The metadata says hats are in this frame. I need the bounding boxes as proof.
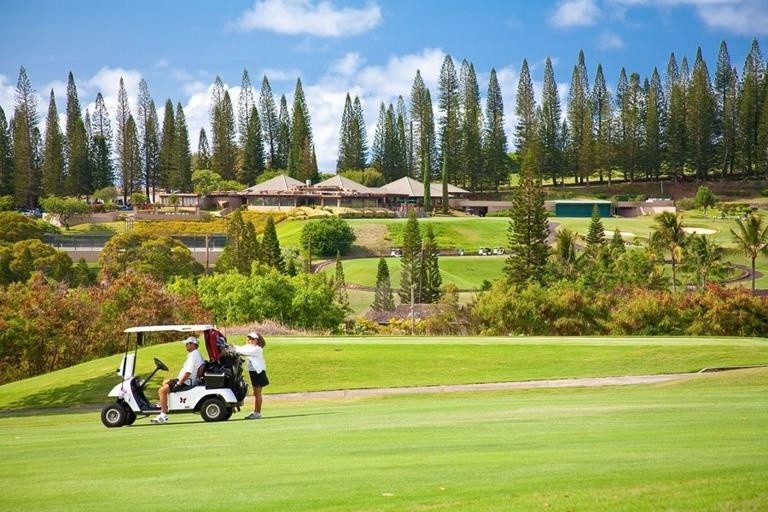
[89,198,132,209]
[21,205,46,219]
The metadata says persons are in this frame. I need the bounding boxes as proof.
[150,336,204,424]
[229,332,269,420]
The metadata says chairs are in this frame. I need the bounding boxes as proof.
[244,412,261,419]
[150,414,168,424]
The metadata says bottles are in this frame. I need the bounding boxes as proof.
[247,331,259,339]
[181,336,199,346]
